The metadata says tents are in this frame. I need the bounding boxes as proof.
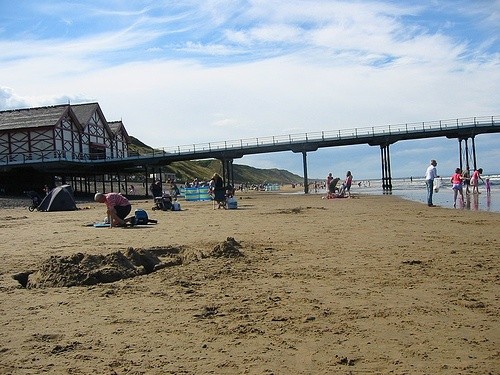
[38,185,77,212]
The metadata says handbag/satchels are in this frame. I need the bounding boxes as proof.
[435,183,439,193]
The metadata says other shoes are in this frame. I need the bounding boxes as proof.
[429,204,436,207]
[118,222,127,227]
[130,216,136,227]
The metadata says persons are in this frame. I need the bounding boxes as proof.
[451,168,469,207]
[326,173,333,192]
[345,171,353,199]
[340,183,347,195]
[185,177,209,188]
[94,192,131,229]
[424,159,440,207]
[464,166,471,194]
[210,173,227,210]
[169,181,180,201]
[485,178,490,193]
[470,168,484,194]
[328,178,340,193]
